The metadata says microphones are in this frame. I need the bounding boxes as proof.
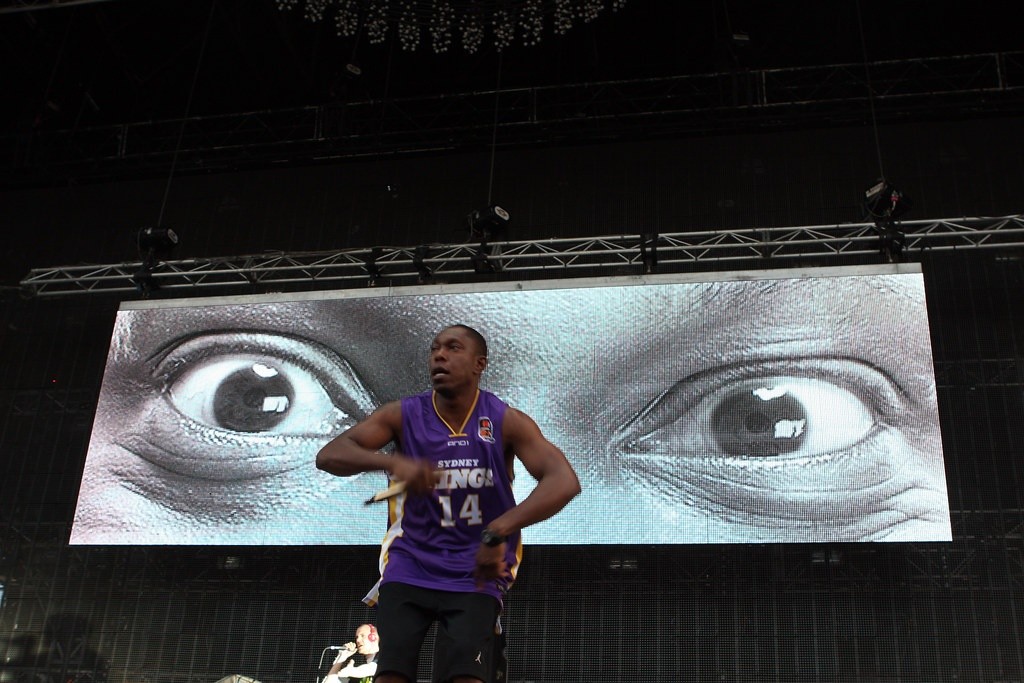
[364,480,408,505]
[326,646,348,650]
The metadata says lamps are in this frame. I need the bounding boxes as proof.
[861,176,911,255]
[136,225,178,267]
[466,205,510,243]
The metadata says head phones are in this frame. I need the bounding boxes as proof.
[368,624,377,642]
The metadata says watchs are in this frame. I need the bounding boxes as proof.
[480,526,506,547]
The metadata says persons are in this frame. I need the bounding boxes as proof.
[316,325,581,683]
[68,262,953,544]
